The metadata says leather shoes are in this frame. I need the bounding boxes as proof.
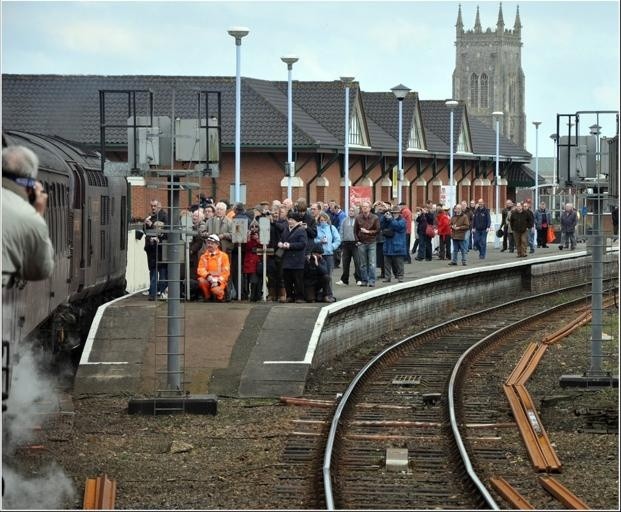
[399,278,403,282]
[383,278,391,282]
[415,257,423,260]
[369,283,375,286]
[361,281,367,286]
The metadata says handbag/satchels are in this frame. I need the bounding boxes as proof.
[547,227,556,243]
[426,224,435,237]
[225,279,237,301]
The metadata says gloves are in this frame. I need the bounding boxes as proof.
[278,241,284,249]
[284,242,290,250]
[207,274,218,283]
[211,282,219,288]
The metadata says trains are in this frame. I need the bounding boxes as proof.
[1,131,131,413]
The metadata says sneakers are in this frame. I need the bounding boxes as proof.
[336,280,347,286]
[448,262,457,265]
[501,248,506,252]
[356,280,362,286]
[143,290,150,296]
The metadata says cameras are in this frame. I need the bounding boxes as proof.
[386,212,390,215]
[17,177,43,190]
[265,210,273,216]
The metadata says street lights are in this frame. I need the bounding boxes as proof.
[279,56,298,200]
[491,111,504,224]
[389,83,412,203]
[548,133,560,219]
[443,101,459,218]
[339,76,355,217]
[224,26,250,205]
[590,124,602,178]
[531,121,543,211]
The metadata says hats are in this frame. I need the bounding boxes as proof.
[392,206,401,212]
[208,234,221,245]
[288,212,299,222]
[253,204,263,214]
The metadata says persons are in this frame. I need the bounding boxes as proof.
[334,198,412,286]
[142,199,169,301]
[180,192,342,302]
[560,203,580,252]
[533,201,552,248]
[410,199,491,265]
[2,147,55,290]
[500,200,535,259]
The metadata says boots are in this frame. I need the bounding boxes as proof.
[268,287,276,301]
[280,287,286,302]
